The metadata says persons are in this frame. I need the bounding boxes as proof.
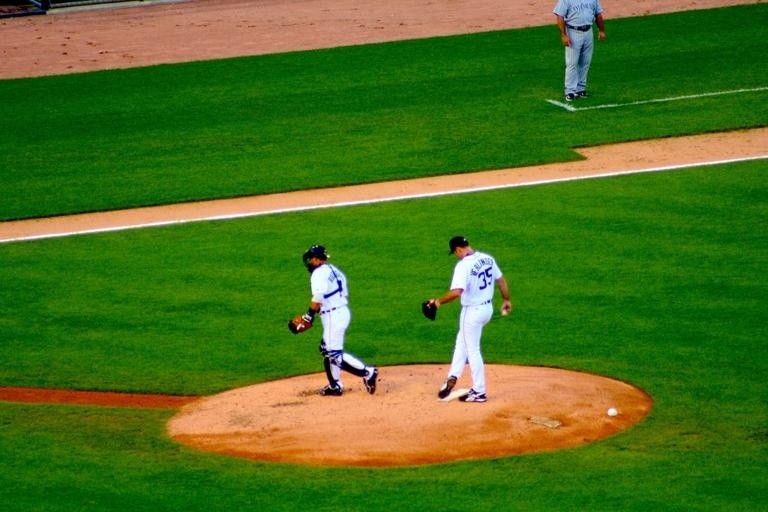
[421,236,512,403]
[288,244,379,396]
[552,0,608,102]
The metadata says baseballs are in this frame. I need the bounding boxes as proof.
[608,408,617,417]
[502,310,508,316]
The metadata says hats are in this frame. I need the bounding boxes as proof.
[447,236,468,255]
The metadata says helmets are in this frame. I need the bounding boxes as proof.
[303,245,327,272]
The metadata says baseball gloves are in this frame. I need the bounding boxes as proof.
[422,298,440,320]
[288,314,313,334]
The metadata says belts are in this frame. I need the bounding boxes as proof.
[565,23,590,32]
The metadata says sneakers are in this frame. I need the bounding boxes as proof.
[459,388,487,402]
[320,384,343,395]
[565,91,590,102]
[439,375,457,399]
[363,366,378,394]
